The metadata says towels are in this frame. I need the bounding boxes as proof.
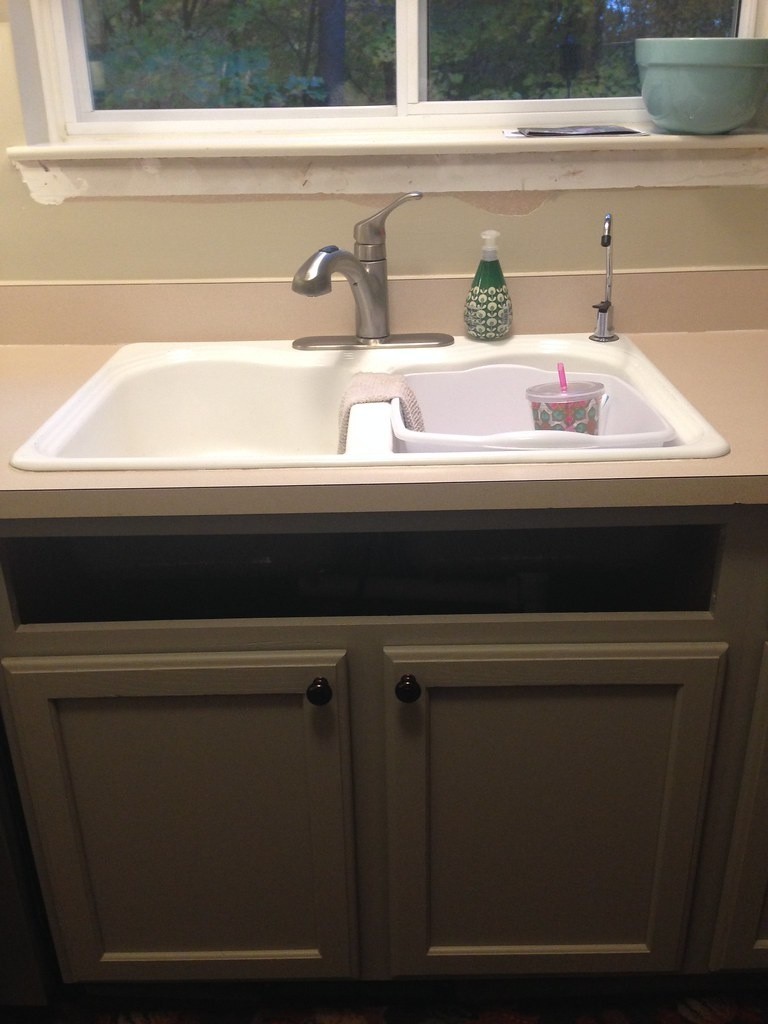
[336,371,427,455]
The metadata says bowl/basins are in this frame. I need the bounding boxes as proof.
[633,36,768,136]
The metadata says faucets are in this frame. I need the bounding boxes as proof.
[590,211,617,338]
[292,191,424,348]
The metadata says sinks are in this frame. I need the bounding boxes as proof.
[51,354,357,460]
[389,349,690,456]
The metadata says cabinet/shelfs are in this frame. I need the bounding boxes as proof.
[1,506,767,991]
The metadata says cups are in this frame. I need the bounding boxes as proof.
[526,378,610,440]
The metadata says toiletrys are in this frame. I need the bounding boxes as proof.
[461,227,514,341]
